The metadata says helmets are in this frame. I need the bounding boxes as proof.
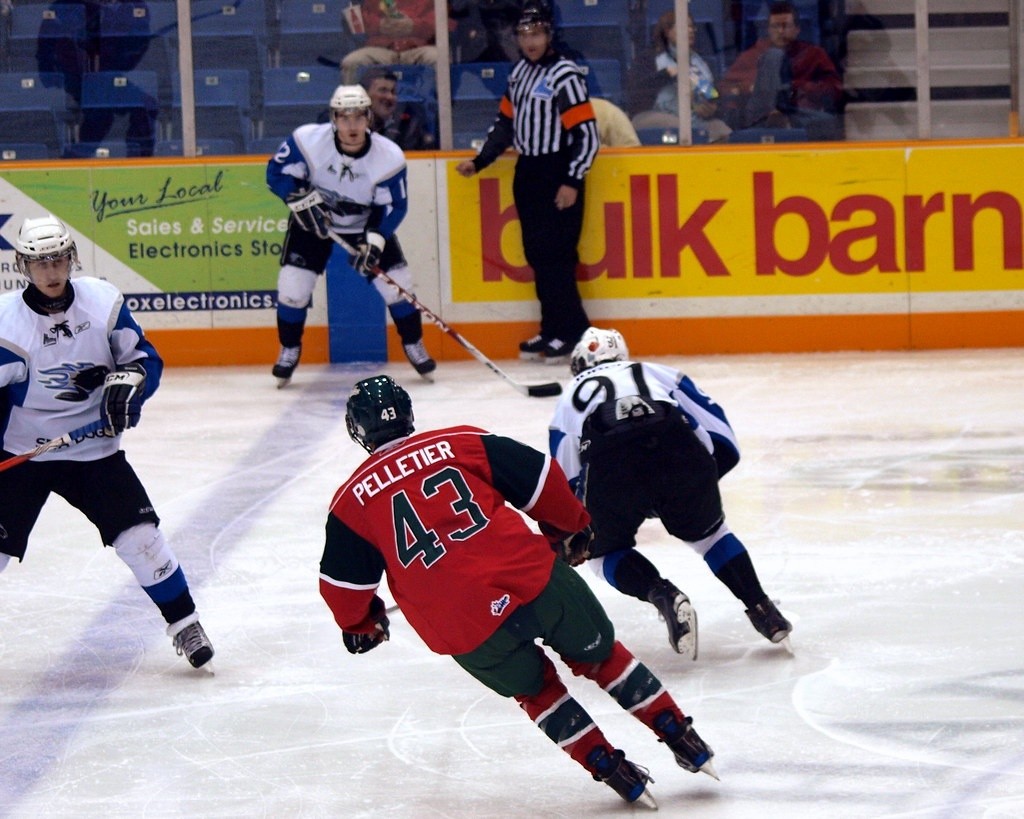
[509,1,552,32]
[330,84,372,108]
[14,215,75,261]
[570,327,629,376]
[345,375,414,456]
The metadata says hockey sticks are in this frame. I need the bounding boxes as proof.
[386,605,399,614]
[0,418,108,471]
[327,227,563,398]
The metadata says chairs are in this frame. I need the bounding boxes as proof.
[0,0,842,163]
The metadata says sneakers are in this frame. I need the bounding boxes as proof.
[545,337,577,365]
[648,579,699,661]
[742,597,795,655]
[402,338,437,383]
[518,334,553,358]
[165,610,216,678]
[653,707,721,781]
[596,753,657,811]
[272,342,303,389]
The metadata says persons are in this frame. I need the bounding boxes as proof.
[0,214,216,674]
[586,97,642,149]
[317,375,719,810]
[457,3,600,366]
[547,327,794,659]
[340,0,457,82]
[626,12,732,142]
[266,86,437,387]
[718,5,848,130]
[318,69,437,152]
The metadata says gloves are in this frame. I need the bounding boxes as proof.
[342,595,391,655]
[99,362,146,437]
[538,523,595,565]
[287,189,334,239]
[349,233,387,279]
